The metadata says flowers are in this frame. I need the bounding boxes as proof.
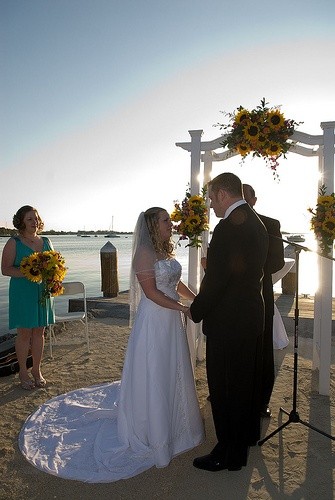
[306,183,335,257]
[19,250,69,305]
[211,95,306,185]
[169,180,211,250]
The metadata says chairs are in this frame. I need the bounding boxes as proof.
[47,281,91,358]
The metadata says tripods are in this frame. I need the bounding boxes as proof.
[257,234,335,446]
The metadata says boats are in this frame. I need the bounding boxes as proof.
[94,236,98,237]
[77,235,91,237]
[287,235,307,242]
[125,237,128,238]
[104,232,120,238]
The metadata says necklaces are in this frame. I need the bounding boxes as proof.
[23,235,39,243]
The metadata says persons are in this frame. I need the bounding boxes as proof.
[185,171,272,473]
[1,205,55,390]
[201,183,285,417]
[116,206,206,470]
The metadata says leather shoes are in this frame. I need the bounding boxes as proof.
[193,454,242,472]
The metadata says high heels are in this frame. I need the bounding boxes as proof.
[18,369,47,389]
